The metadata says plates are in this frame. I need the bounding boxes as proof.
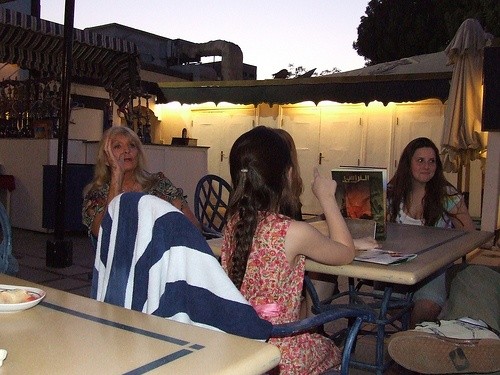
[0,283,47,312]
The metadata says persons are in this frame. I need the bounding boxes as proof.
[387,265,500,375]
[219,126,383,375]
[305,138,476,375]
[82,126,202,255]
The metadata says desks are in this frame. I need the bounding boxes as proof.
[0,273,281,375]
[204,218,495,375]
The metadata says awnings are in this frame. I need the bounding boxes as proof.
[156,70,454,106]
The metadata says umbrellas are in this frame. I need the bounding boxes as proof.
[440,19,492,197]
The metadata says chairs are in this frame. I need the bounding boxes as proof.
[90,174,470,375]
[0,201,19,277]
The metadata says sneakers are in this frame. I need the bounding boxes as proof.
[385,319,500,374]
